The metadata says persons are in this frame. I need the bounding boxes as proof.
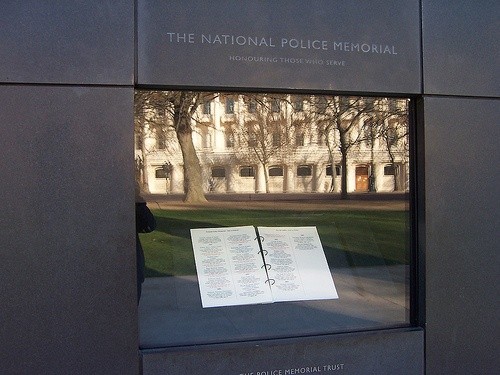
[136,181,157,308]
